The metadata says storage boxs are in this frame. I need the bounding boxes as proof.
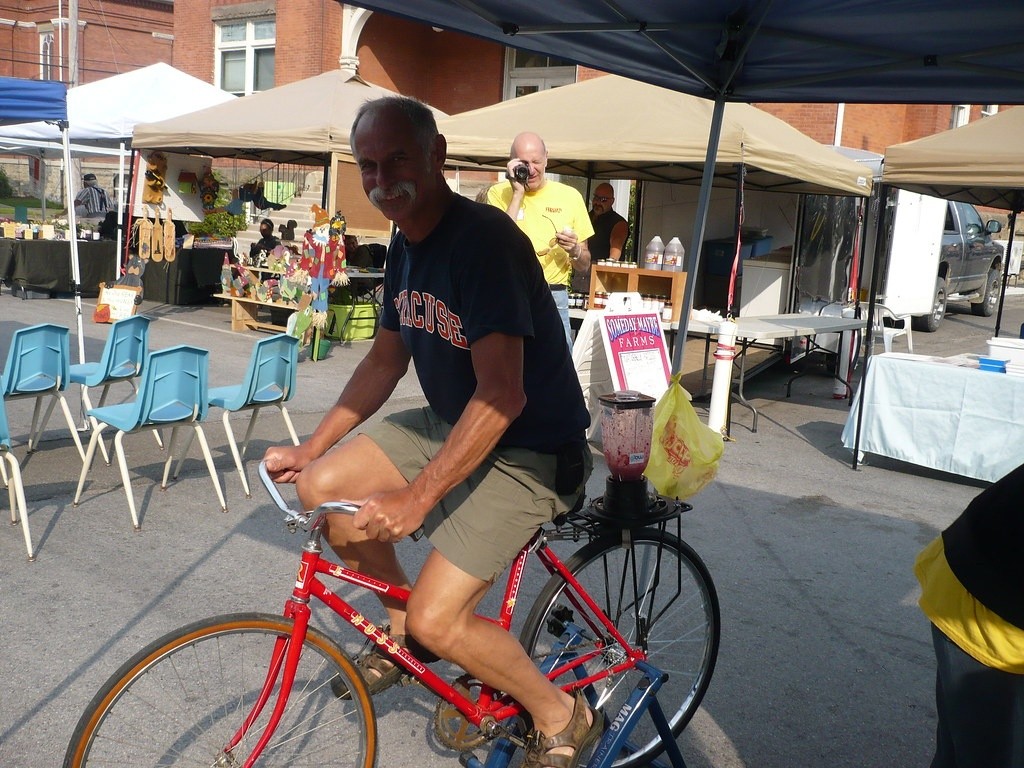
[732,234,773,259]
[326,302,379,339]
[704,239,753,278]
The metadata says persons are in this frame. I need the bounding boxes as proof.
[60,174,111,217]
[264,97,604,768]
[487,132,595,354]
[912,463,1024,768]
[250,219,281,257]
[587,184,630,261]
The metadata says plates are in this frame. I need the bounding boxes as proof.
[351,268,360,271]
[367,268,380,273]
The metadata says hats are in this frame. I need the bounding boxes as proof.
[81,173,96,181]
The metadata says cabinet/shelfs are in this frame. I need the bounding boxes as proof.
[586,263,687,322]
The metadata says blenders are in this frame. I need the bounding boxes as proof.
[598,390,658,512]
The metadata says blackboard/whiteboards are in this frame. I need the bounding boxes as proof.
[98,282,143,324]
[618,346,669,408]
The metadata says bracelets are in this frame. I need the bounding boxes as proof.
[572,245,581,260]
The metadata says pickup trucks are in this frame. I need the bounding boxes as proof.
[865,200,1004,334]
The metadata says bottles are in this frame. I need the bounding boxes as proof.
[86,222,92,241]
[662,235,685,272]
[640,294,668,315]
[33,226,39,239]
[595,291,611,308]
[568,292,589,310]
[597,258,638,269]
[661,303,672,323]
[644,234,665,270]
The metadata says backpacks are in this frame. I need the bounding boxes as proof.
[363,243,387,268]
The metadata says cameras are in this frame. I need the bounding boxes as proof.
[514,160,529,182]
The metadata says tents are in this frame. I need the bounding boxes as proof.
[0,73,90,430]
[118,68,871,439]
[0,62,238,281]
[853,105,1024,469]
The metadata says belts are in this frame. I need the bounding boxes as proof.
[568,429,585,438]
[548,284,567,291]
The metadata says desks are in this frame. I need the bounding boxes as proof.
[670,313,868,433]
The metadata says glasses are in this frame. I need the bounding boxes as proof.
[592,193,613,203]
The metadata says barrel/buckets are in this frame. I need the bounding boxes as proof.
[307,338,331,360]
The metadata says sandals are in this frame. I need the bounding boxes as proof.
[520,691,603,768]
[331,629,440,699]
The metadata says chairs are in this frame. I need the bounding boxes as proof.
[74,345,227,532]
[0,376,35,560]
[174,333,301,498]
[858,301,913,353]
[33,315,164,466]
[0,324,85,463]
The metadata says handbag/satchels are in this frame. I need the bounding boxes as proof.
[641,371,726,501]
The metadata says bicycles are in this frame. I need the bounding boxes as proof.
[58,459,722,768]
